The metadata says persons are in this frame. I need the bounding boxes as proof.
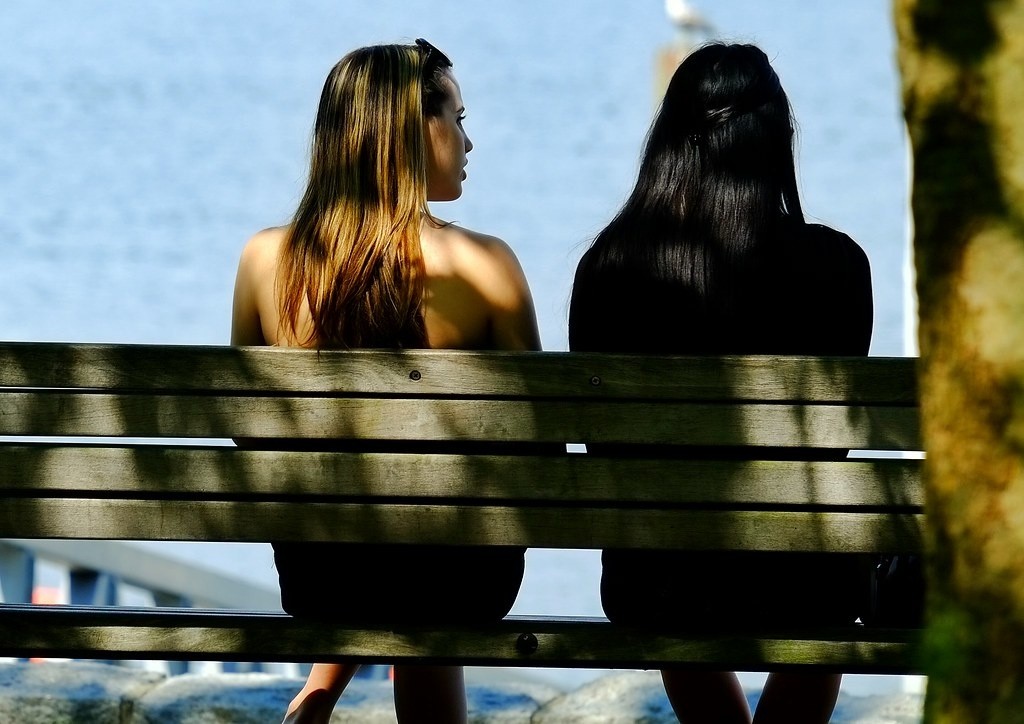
[569,43,874,724]
[227,41,542,724]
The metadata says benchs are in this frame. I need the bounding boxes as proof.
[0,342,927,674]
